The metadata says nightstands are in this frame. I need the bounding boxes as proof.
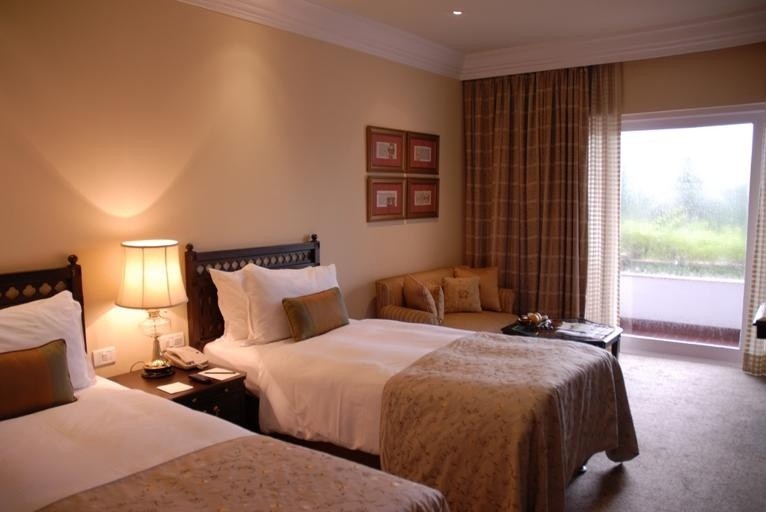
[107,352,247,431]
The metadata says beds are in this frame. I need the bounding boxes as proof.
[184,233,639,511]
[1,254,455,512]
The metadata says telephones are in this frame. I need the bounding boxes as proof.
[164,346,209,370]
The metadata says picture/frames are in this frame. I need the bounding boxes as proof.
[406,130,440,174]
[408,178,439,219]
[365,125,406,172]
[367,176,407,222]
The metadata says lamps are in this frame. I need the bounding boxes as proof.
[113,239,189,381]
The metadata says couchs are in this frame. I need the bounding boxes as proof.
[375,264,520,339]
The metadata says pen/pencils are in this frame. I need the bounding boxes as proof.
[205,372,235,374]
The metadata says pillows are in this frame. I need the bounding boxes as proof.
[304,263,341,296]
[453,266,502,312]
[239,265,318,347]
[282,286,350,342]
[2,289,96,391]
[403,273,438,322]
[441,277,481,313]
[207,268,246,341]
[1,338,77,421]
[429,285,444,320]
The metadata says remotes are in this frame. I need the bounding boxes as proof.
[188,373,213,384]
